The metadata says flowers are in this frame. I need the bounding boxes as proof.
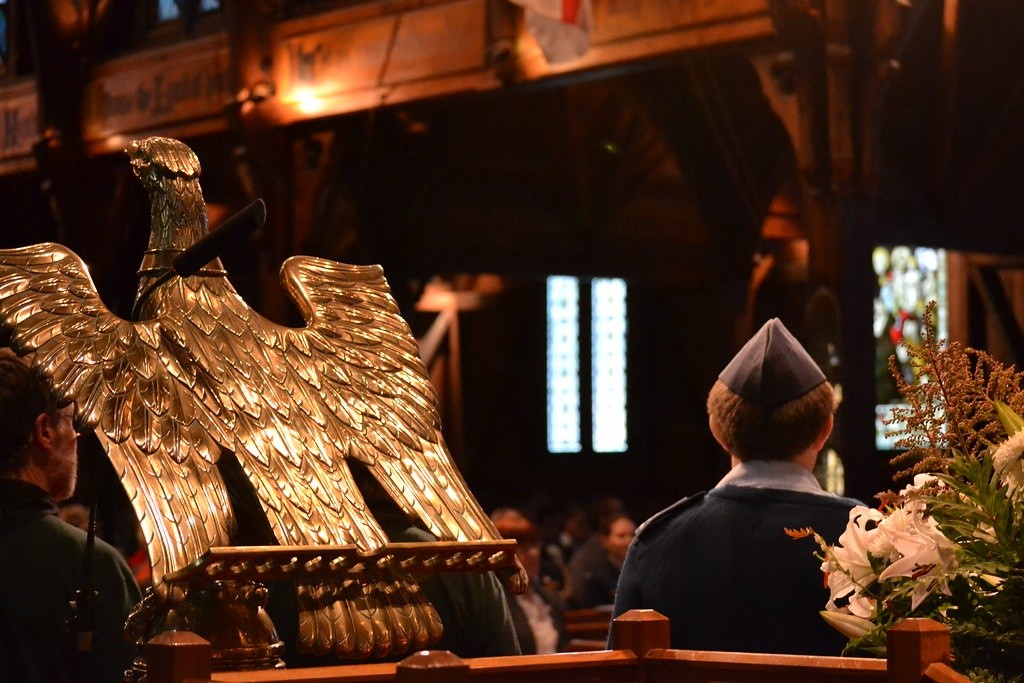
[806,400,1024,654]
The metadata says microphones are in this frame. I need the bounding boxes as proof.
[58,199,266,683]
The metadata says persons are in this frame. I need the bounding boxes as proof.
[402,498,640,655]
[606,317,890,658]
[0,346,144,683]
[57,503,152,593]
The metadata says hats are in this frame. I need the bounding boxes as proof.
[719,317,827,411]
